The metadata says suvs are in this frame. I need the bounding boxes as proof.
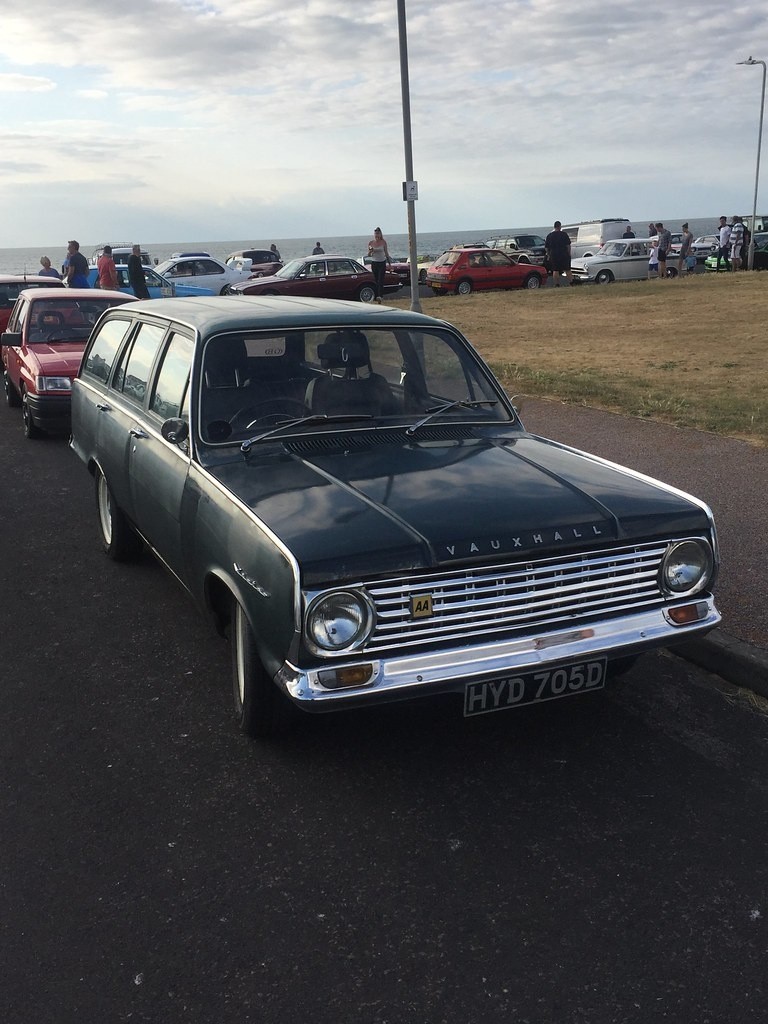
[484,233,546,266]
[68,293,726,742]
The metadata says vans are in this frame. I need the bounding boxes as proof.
[552,218,636,258]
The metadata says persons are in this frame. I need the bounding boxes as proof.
[469,254,487,267]
[98,245,120,291]
[655,223,672,279]
[622,226,636,239]
[39,256,61,280]
[172,262,192,275]
[716,216,749,274]
[128,244,153,299]
[648,241,658,279]
[545,221,576,288]
[677,223,697,279]
[271,244,281,259]
[62,241,90,288]
[313,242,325,255]
[369,227,392,304]
[648,223,657,237]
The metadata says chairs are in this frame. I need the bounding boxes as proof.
[478,257,486,265]
[30,311,64,341]
[204,344,260,424]
[305,328,398,413]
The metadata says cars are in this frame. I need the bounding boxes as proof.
[648,215,768,271]
[86,246,213,281]
[0,287,140,440]
[0,273,90,335]
[424,248,548,296]
[62,263,218,299]
[561,238,688,285]
[230,242,506,304]
[144,256,257,296]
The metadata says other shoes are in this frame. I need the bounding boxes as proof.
[377,297,382,303]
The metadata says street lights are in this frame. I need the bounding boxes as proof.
[734,54,767,271]
